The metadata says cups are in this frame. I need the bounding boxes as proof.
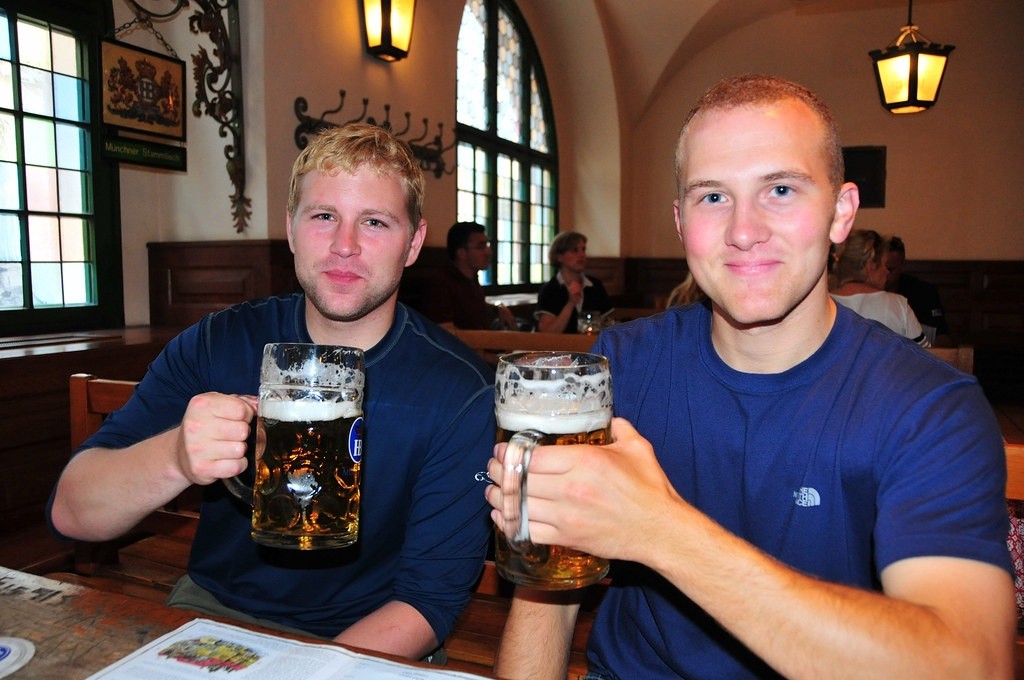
[578,311,603,337]
[493,349,615,604]
[221,342,366,551]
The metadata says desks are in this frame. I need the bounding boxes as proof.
[0,565,507,680]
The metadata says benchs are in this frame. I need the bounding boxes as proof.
[441,322,975,375]
[45,366,1024,680]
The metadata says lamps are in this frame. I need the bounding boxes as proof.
[867,0,955,114]
[358,0,417,63]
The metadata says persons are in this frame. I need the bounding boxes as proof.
[486,75,1019,680]
[534,231,610,335]
[50,124,498,668]
[826,229,936,348]
[430,221,518,332]
